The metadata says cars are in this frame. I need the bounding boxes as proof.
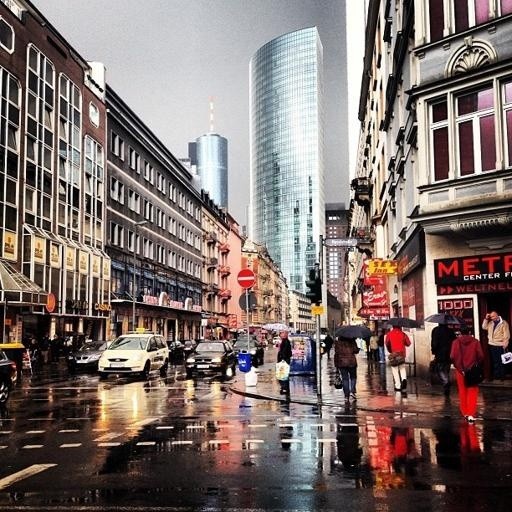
[0,350,18,404]
[167,340,264,378]
[68,341,111,370]
[98,334,169,378]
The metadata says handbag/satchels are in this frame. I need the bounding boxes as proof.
[500,347,512,365]
[463,362,485,387]
[334,371,342,389]
[275,359,291,381]
[388,351,405,366]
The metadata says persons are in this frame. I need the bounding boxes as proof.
[333,336,360,403]
[27,332,73,377]
[276,332,292,394]
[482,311,510,384]
[362,325,412,398]
[430,322,457,387]
[323,333,334,360]
[449,325,485,422]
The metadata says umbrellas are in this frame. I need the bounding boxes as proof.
[388,317,421,329]
[261,323,294,331]
[336,326,374,338]
[423,314,466,326]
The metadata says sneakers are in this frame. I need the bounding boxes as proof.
[344,397,349,402]
[467,415,474,421]
[349,392,356,399]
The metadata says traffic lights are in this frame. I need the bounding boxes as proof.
[306,269,322,303]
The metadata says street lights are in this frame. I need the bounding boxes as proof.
[132,220,146,330]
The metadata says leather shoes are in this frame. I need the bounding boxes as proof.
[401,379,407,389]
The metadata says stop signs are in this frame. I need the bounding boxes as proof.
[237,269,256,289]
